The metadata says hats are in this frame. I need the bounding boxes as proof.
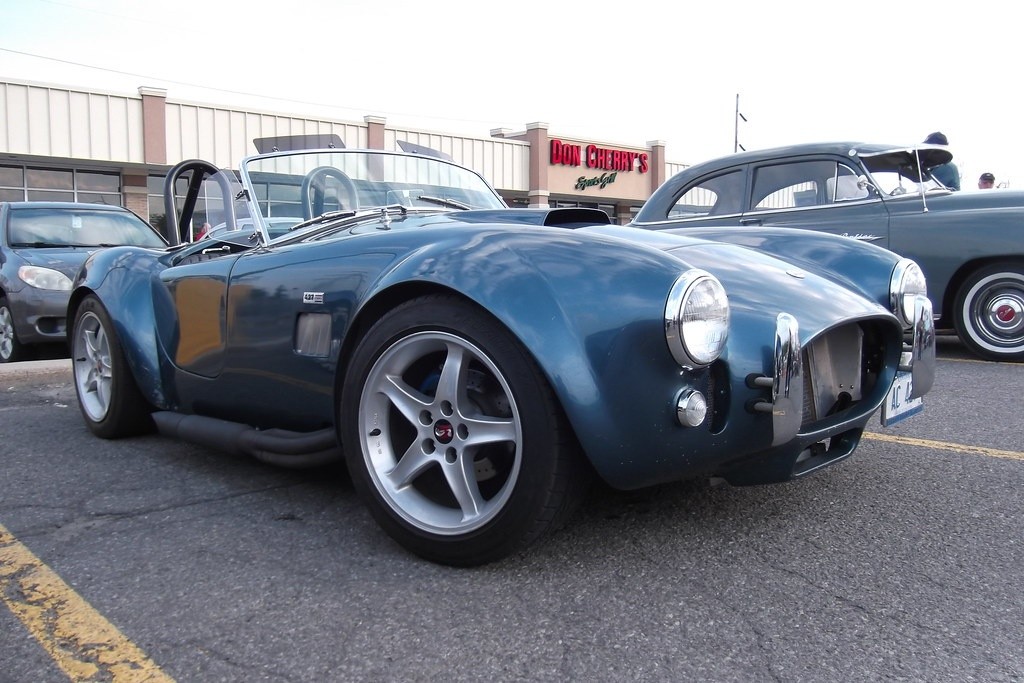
[980,173,995,181]
[923,132,948,145]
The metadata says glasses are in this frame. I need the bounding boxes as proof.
[982,181,994,184]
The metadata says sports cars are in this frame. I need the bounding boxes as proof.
[66,142,936,569]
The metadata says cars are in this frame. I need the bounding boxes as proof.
[0,202,173,363]
[622,142,1024,364]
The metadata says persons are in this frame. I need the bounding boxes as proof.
[978,173,995,189]
[922,131,960,191]
[776,332,792,399]
[195,223,212,241]
